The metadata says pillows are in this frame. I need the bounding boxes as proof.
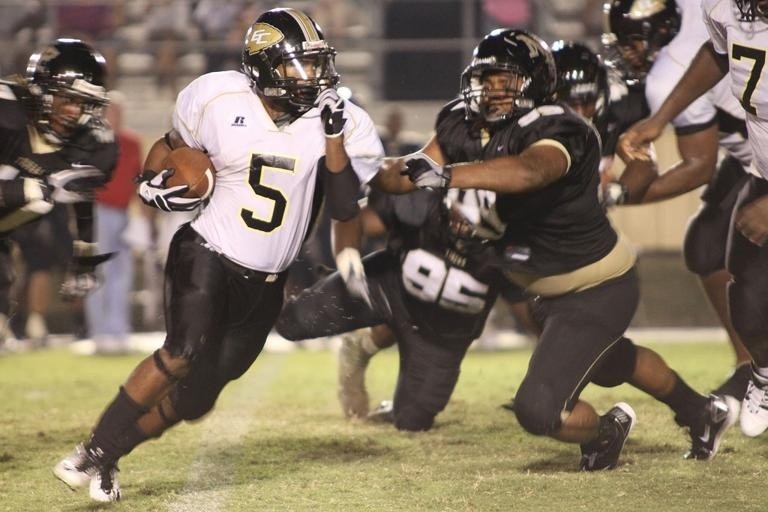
[459,29,557,132]
[241,6,340,115]
[602,1,682,67]
[553,41,607,121]
[733,0,768,24]
[27,37,111,150]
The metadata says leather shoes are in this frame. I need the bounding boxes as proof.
[398,152,452,194]
[337,247,376,312]
[135,169,203,212]
[597,179,628,215]
[314,87,347,138]
[59,240,107,302]
[47,165,106,205]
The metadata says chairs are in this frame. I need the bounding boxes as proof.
[683,389,740,461]
[52,438,121,502]
[674,361,768,438]
[580,401,635,471]
[334,328,394,423]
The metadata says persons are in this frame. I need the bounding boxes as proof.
[52,5,383,503]
[380,27,740,474]
[0,2,269,353]
[276,113,536,432]
[547,1,767,439]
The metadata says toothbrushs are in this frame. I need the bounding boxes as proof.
[159,147,217,203]
